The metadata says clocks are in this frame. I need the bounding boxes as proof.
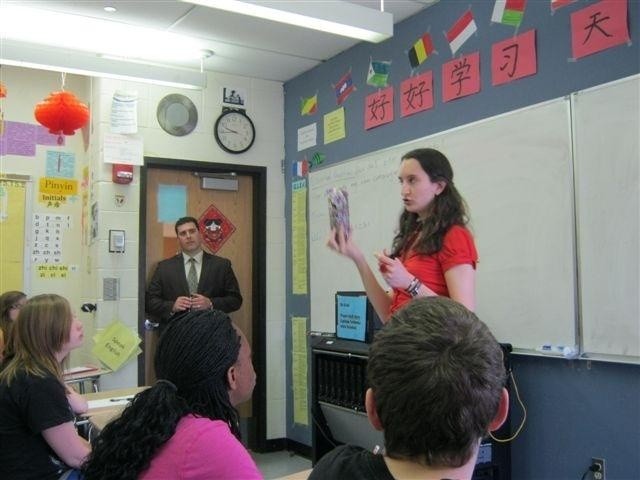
[213,110,256,154]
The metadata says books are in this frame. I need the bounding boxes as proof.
[326,187,355,242]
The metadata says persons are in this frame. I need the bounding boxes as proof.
[1,294,95,480]
[326,148,477,325]
[1,291,26,342]
[145,217,243,339]
[306,296,513,480]
[1,320,88,414]
[78,309,266,479]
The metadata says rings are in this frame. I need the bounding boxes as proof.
[197,305,201,308]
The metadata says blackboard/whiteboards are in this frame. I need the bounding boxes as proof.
[306,75,575,366]
[568,72,640,367]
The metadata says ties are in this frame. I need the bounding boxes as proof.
[186,257,199,300]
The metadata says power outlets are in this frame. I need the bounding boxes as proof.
[591,457,606,480]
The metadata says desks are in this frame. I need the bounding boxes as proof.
[80,385,157,441]
[64,375,100,394]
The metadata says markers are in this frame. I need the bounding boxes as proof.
[535,344,572,355]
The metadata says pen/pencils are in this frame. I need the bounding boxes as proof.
[110,398,133,401]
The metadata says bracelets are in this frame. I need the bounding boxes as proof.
[404,275,423,296]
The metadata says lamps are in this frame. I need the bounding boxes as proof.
[1,35,214,91]
[182,0,395,44]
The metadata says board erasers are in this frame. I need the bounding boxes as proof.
[499,342,513,352]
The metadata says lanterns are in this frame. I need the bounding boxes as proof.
[35,91,90,146]
